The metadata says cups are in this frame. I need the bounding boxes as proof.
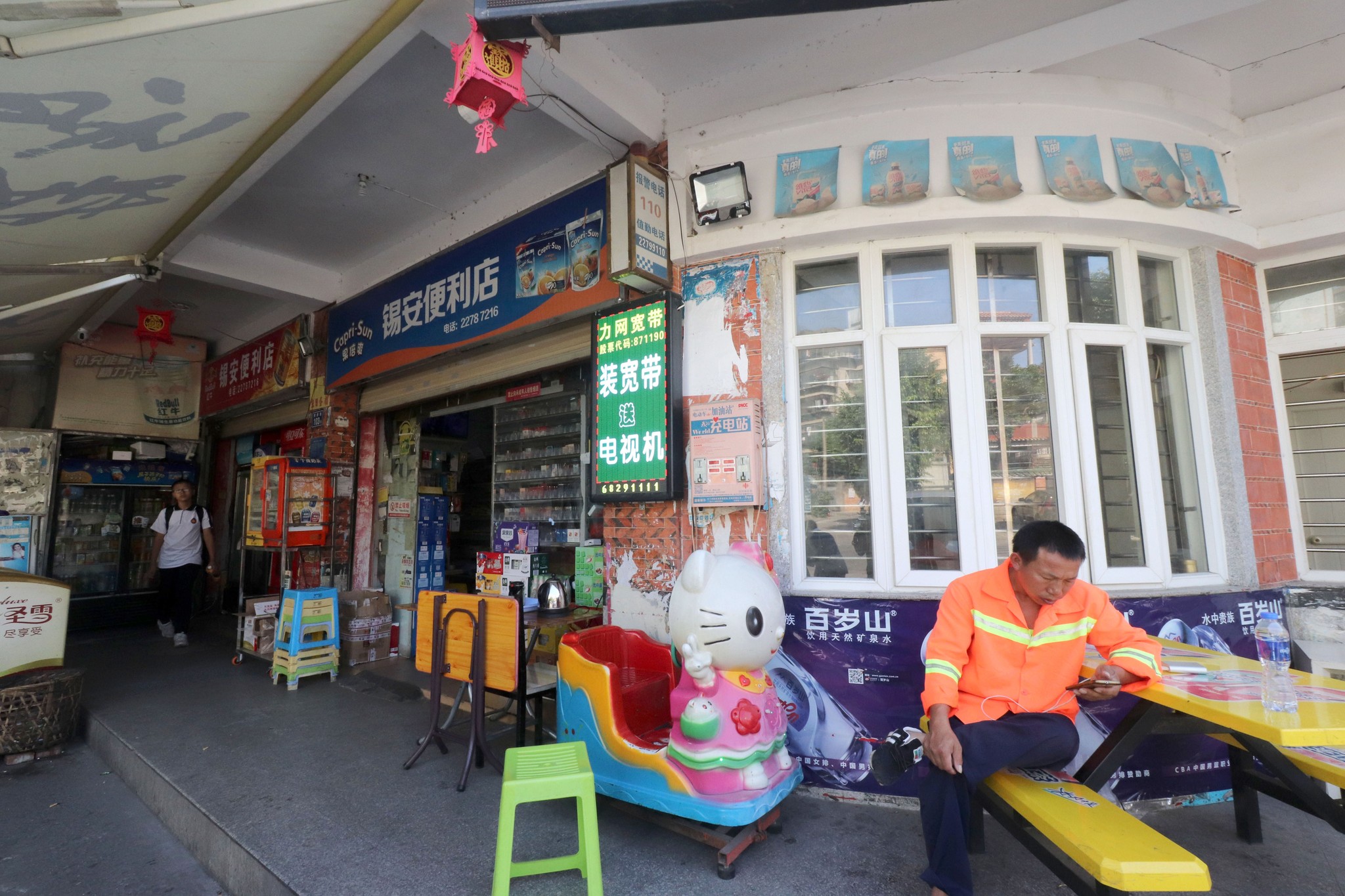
[517,529,528,553]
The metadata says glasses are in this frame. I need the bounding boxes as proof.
[172,488,190,493]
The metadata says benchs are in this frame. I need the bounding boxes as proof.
[1205,734,1345,790]
[920,716,1212,896]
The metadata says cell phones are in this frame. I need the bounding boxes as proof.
[1065,680,1120,690]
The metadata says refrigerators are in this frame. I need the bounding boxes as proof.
[0,565,71,678]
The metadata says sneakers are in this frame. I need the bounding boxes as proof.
[869,726,926,786]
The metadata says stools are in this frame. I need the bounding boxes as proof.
[491,741,604,896]
[271,586,341,690]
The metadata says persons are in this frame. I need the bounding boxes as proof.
[803,516,876,580]
[146,478,217,646]
[917,519,1165,895]
[11,543,25,560]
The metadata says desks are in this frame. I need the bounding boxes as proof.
[404,590,519,791]
[1073,634,1345,845]
[396,603,603,746]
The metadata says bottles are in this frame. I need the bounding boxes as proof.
[208,566,220,582]
[132,498,162,514]
[1253,612,1298,714]
[69,493,116,514]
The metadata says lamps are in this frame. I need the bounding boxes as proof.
[298,336,315,359]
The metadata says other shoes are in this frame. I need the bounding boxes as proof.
[174,632,189,648]
[158,618,174,639]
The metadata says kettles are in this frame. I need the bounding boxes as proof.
[537,574,570,619]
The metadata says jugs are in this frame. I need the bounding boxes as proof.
[508,581,525,605]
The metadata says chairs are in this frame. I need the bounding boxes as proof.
[484,600,557,745]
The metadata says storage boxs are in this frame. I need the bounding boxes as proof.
[411,497,448,658]
[575,547,603,608]
[476,521,548,598]
[230,596,279,654]
[556,529,580,542]
[338,590,392,667]
